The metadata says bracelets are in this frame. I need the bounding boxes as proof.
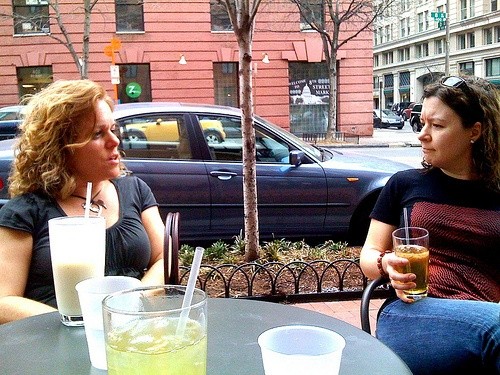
[376,249,391,278]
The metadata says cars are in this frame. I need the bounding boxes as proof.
[392,102,425,134]
[373,109,404,130]
[119,114,226,145]
[0,105,418,248]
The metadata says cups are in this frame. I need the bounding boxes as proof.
[73,274,143,371]
[391,227,429,302]
[101,285,208,375]
[48,215,107,327]
[257,325,346,375]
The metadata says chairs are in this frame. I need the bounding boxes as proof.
[359,274,397,334]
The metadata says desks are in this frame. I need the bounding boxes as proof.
[0,297,414,375]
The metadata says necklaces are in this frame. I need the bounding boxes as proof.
[71,189,106,213]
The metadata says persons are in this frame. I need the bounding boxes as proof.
[360,73,500,375]
[0,79,172,323]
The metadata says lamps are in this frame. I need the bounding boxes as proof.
[178,54,187,65]
[261,53,270,63]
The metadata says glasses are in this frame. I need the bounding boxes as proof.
[436,75,473,99]
